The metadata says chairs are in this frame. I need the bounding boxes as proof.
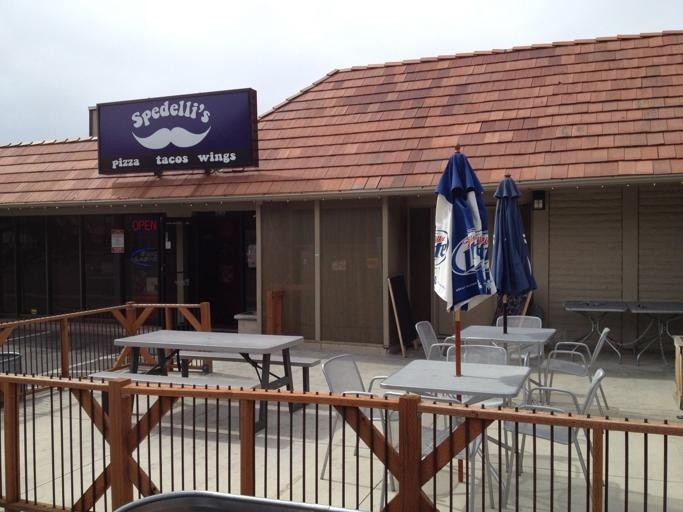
[412,319,443,360]
[378,390,498,510]
[501,365,609,510]
[538,326,611,417]
[443,343,510,366]
[494,316,543,404]
[318,352,398,494]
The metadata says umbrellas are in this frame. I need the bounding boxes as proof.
[490,169,538,333]
[431,143,498,377]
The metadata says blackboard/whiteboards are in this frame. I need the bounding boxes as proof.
[389,273,419,345]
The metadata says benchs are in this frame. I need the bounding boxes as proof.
[89,373,267,437]
[174,349,317,411]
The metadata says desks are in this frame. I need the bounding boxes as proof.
[450,324,558,411]
[563,296,683,368]
[87,327,320,435]
[380,358,533,506]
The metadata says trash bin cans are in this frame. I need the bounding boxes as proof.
[0,352,22,404]
